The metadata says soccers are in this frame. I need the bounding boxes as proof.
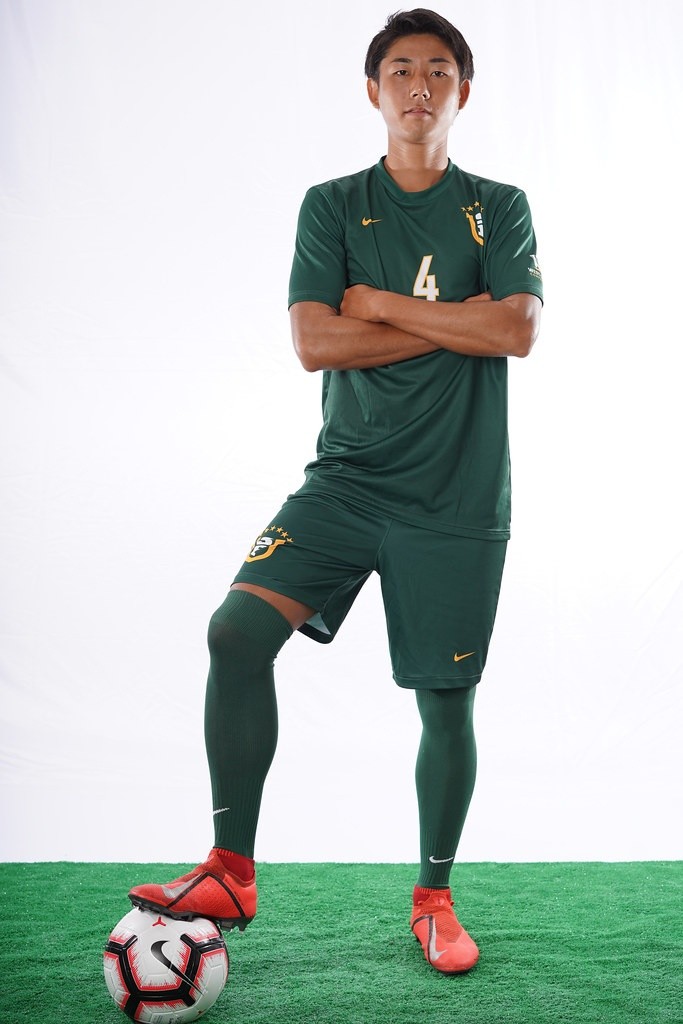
[103,905,228,1023]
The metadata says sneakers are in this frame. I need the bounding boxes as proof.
[128,860,261,932]
[411,887,479,973]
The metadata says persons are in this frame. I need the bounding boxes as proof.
[126,6,545,976]
